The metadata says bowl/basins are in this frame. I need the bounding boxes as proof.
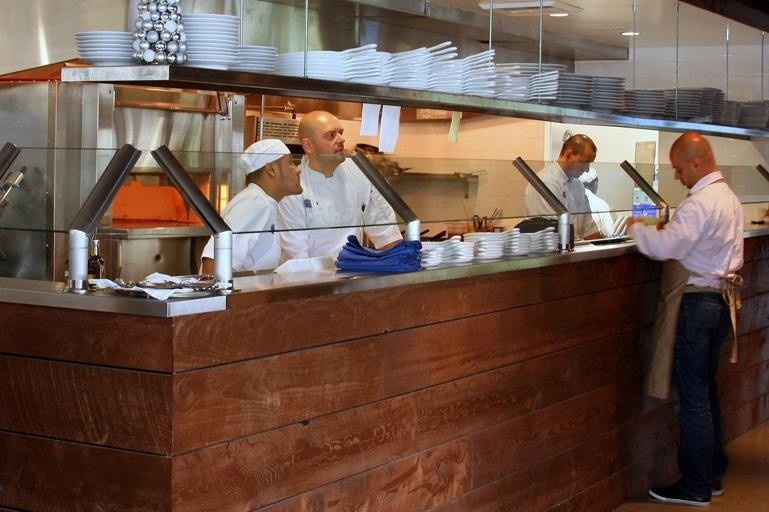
[177,11,278,72]
[74,29,144,66]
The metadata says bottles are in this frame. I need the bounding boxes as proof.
[88,238,106,280]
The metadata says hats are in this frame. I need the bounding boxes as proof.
[238,138,292,176]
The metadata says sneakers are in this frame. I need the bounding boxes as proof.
[649,476,723,507]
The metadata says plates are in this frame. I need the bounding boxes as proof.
[558,70,768,132]
[274,41,568,102]
[418,226,562,271]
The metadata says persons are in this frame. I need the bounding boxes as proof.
[578,167,619,240]
[196,138,305,277]
[625,130,749,506]
[275,110,404,263]
[525,133,614,244]
